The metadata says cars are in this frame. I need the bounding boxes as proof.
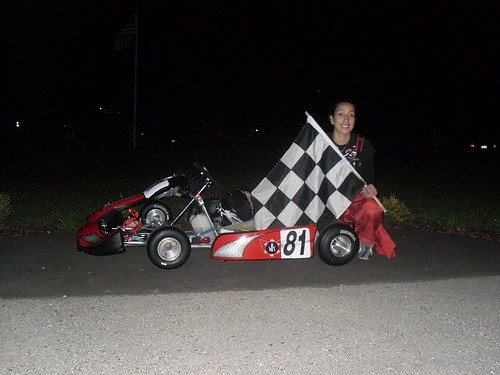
[467,132,500,153]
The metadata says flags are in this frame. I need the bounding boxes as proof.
[247,116,366,245]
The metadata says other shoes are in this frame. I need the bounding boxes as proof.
[359,246,372,260]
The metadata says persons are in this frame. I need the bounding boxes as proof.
[322,100,384,260]
